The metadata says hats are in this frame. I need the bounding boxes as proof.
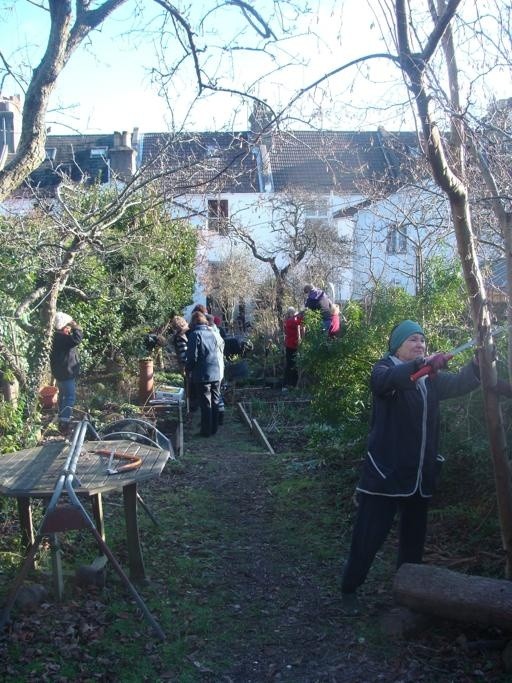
[192,303,207,313]
[213,315,221,326]
[303,284,314,293]
[190,311,208,324]
[54,311,73,331]
[171,315,188,331]
[205,314,214,325]
[330,303,340,314]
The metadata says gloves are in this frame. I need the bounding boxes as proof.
[423,351,454,373]
[473,343,499,365]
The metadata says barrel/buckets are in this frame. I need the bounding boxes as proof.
[38,386,59,408]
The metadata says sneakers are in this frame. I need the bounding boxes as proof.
[340,589,362,617]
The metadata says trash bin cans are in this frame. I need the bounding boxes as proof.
[226,359,250,380]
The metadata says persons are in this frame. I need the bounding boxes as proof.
[337,317,498,601]
[48,311,84,425]
[296,282,345,344]
[171,303,227,437]
[281,304,298,391]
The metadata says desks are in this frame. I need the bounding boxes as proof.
[1,439,172,634]
